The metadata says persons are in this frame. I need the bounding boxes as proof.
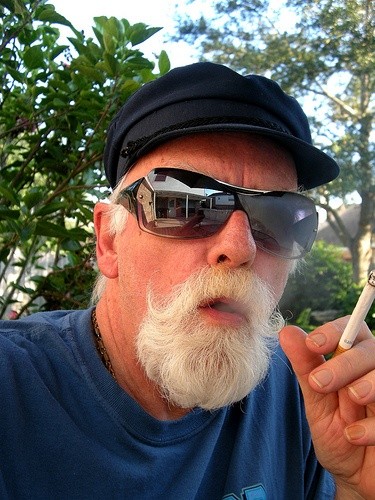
[0,61,375,500]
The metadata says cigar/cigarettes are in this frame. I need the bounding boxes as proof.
[331,271,375,359]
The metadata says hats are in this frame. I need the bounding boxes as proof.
[104,63,340,190]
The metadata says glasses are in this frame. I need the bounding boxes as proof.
[117,166,321,259]
